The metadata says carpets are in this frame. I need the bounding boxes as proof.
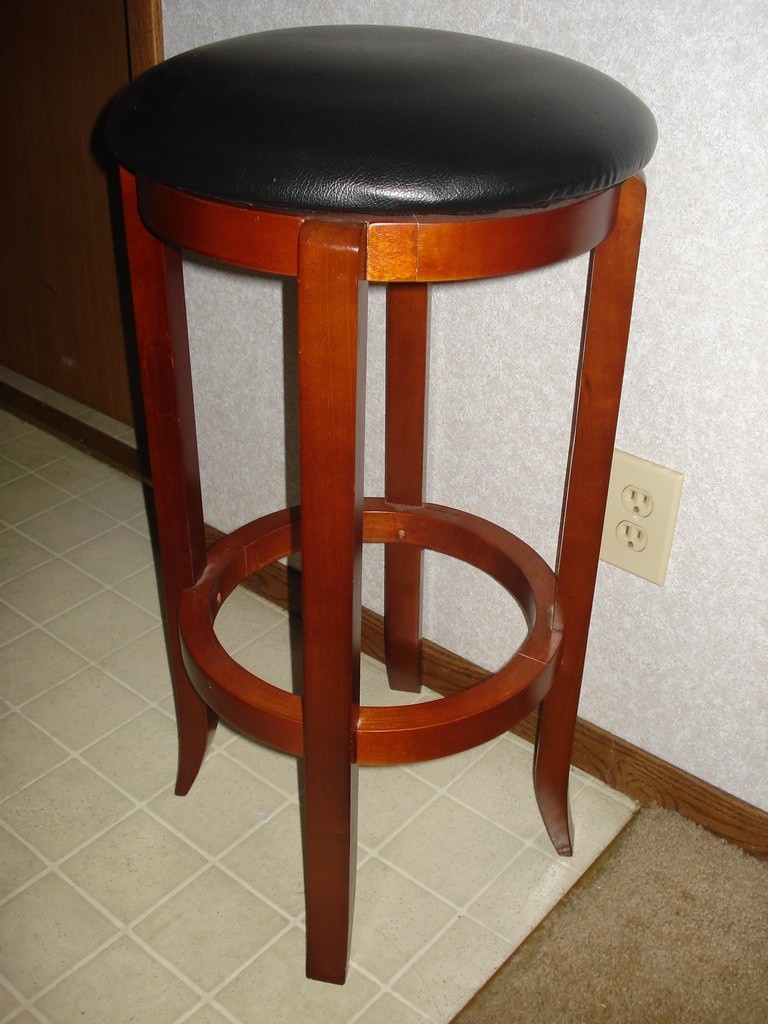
[444,796,768,1024]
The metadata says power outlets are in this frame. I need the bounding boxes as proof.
[597,447,684,586]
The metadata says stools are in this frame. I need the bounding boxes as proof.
[90,25,656,985]
